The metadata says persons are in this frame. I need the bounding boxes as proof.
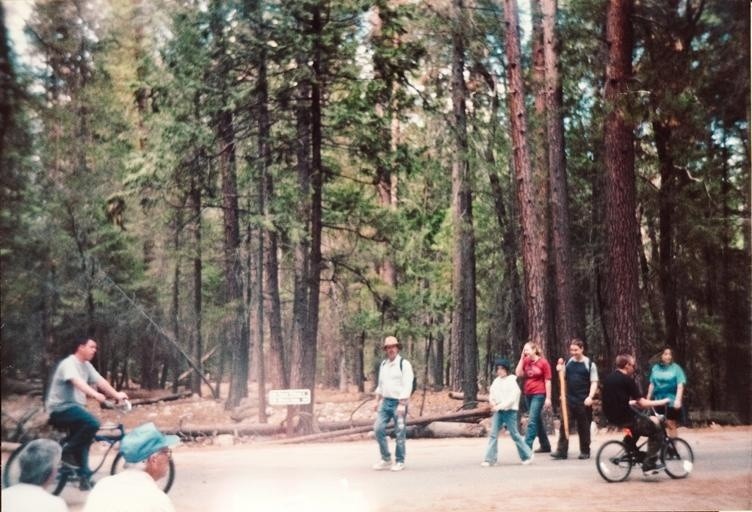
[45,335,129,492]
[480,358,536,468]
[646,344,687,459]
[550,337,600,461]
[601,353,672,476]
[514,340,553,453]
[82,421,182,512]
[1,437,69,512]
[370,335,416,472]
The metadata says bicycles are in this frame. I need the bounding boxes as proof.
[593,399,697,484]
[3,395,177,496]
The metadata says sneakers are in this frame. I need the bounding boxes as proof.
[481,458,498,466]
[533,445,551,452]
[578,451,590,459]
[61,450,82,469]
[373,458,393,470]
[642,459,667,477]
[549,449,567,459]
[519,454,534,465]
[392,461,406,472]
[81,480,96,493]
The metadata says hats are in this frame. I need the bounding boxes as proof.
[494,358,511,376]
[381,335,403,351]
[119,422,181,461]
